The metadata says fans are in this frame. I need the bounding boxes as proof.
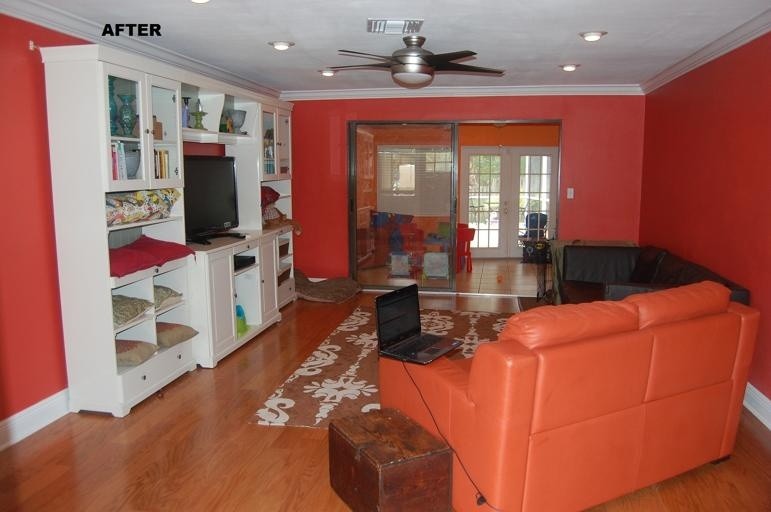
[317,35,504,75]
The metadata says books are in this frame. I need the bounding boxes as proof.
[112,142,128,180]
[154,149,169,179]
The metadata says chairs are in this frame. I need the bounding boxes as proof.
[518,199,543,223]
[359,223,475,288]
[469,197,486,224]
[518,213,547,246]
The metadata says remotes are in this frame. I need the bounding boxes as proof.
[235,235,246,238]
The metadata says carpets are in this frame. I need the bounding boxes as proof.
[247,305,516,428]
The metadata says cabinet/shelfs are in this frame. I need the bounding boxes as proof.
[38,43,295,418]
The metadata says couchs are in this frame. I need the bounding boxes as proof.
[549,237,749,306]
[379,301,762,512]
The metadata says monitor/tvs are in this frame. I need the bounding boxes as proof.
[183,156,241,246]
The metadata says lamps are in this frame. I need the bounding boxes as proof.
[387,63,435,89]
[563,64,576,72]
[583,31,602,41]
[268,41,295,51]
[317,70,338,77]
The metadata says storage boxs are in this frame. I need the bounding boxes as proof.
[328,408,453,512]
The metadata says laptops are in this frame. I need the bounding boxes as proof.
[376,283,464,366]
